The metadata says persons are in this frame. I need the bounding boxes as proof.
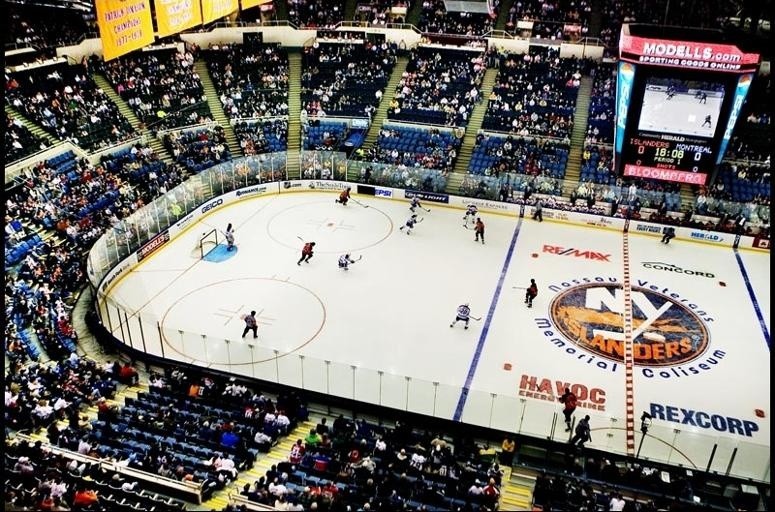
[524,279,538,308]
[1,362,705,512]
[1,0,211,363]
[241,311,258,338]
[450,301,470,329]
[215,0,771,251]
[338,254,356,270]
[297,242,315,265]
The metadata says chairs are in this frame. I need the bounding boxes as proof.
[5,0,772,512]
